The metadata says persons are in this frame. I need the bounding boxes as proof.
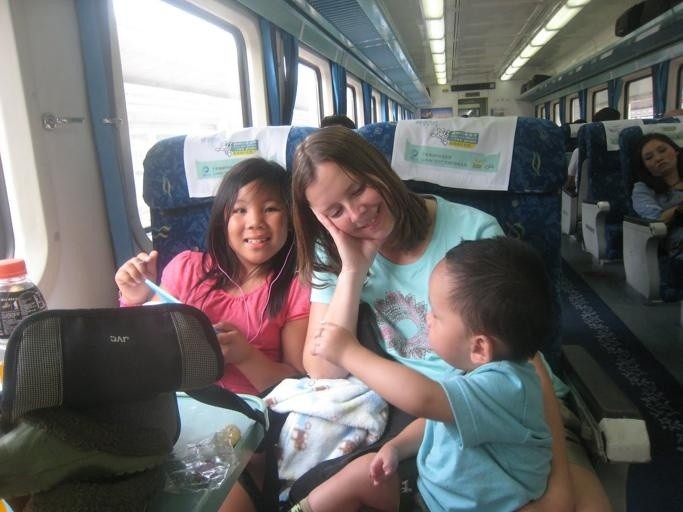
[288,124,618,512]
[110,158,315,511]
[627,129,682,304]
[563,106,622,197]
[274,234,559,512]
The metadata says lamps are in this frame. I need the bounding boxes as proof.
[500,0,591,81]
[419,0,450,86]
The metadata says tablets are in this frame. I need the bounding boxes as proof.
[145,279,223,335]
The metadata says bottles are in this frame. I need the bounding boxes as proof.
[0,258,49,391]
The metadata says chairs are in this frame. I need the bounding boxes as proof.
[565,113,682,303]
[146,118,649,510]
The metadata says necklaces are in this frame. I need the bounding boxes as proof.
[670,181,681,187]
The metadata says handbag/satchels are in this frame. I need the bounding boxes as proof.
[0,303,225,500]
[258,303,418,512]
